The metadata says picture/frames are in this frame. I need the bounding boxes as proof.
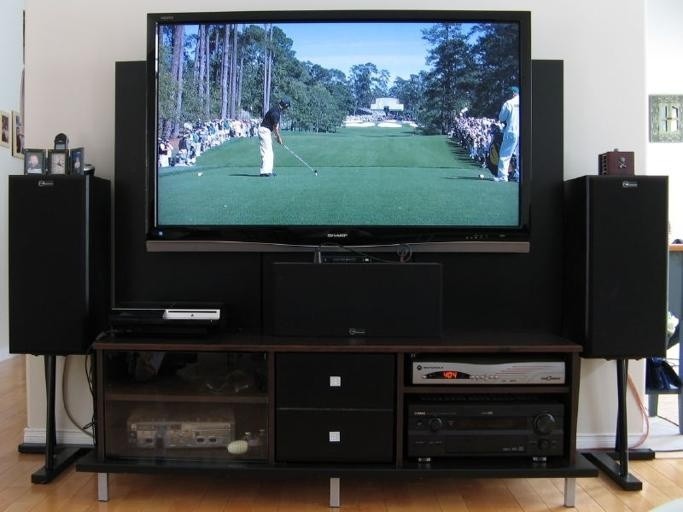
[22,146,45,176]
[69,147,86,175]
[10,110,23,160]
[0,108,11,149]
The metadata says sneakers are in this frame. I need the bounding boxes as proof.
[494,177,508,182]
[260,173,276,177]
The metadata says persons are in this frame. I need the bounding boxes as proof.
[443,105,519,181]
[343,109,420,130]
[490,85,519,182]
[256,97,288,181]
[156,117,285,168]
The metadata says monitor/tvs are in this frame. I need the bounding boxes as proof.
[144,10,531,259]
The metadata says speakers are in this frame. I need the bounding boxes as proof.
[8,176,112,355]
[272,264,448,338]
[562,173,670,359]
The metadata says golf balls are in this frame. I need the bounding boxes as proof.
[480,175,484,179]
[198,172,203,176]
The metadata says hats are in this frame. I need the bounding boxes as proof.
[509,86,519,94]
[282,98,291,107]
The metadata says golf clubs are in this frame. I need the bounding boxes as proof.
[281,144,317,173]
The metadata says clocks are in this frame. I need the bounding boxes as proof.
[46,147,69,174]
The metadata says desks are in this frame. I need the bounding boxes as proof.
[645,240,683,439]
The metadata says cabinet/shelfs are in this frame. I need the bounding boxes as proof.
[71,334,600,509]
